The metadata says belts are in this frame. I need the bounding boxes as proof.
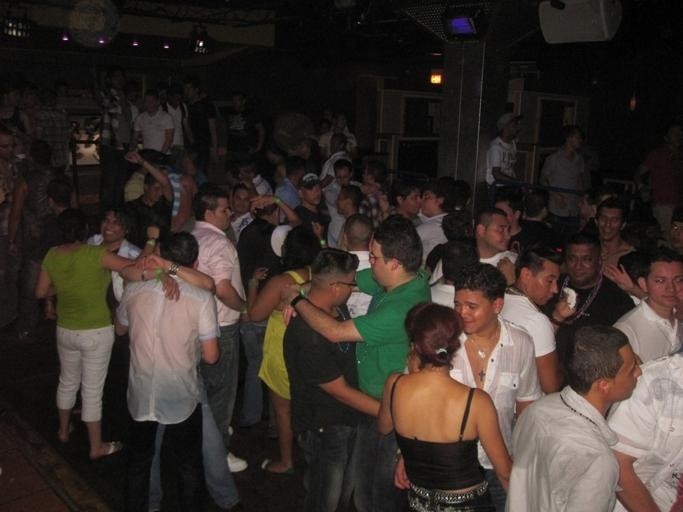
[410,481,489,504]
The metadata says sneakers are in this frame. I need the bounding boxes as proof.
[229,427,233,436]
[227,453,248,472]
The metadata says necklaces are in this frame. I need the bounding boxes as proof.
[464,330,499,382]
[328,305,352,355]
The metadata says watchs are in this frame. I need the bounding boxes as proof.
[167,261,183,275]
[288,295,306,309]
[546,314,564,327]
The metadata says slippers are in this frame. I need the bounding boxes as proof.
[58,425,73,434]
[108,441,123,455]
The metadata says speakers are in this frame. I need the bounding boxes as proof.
[538,0,623,44]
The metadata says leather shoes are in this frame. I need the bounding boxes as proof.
[255,456,295,479]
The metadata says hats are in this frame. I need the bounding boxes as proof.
[497,113,524,132]
[298,173,323,190]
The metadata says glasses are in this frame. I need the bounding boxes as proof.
[1,143,17,150]
[330,280,358,292]
[369,253,396,264]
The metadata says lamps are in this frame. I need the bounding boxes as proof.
[163,41,171,50]
[60,31,70,42]
[196,40,207,56]
[131,38,141,47]
[98,36,105,44]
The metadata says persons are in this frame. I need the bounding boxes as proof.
[132,93,176,157]
[43,207,145,421]
[184,82,219,161]
[175,140,219,198]
[361,172,393,227]
[427,207,521,287]
[117,233,220,510]
[324,159,382,247]
[0,129,24,327]
[153,146,196,231]
[541,232,636,361]
[231,161,276,196]
[502,324,644,512]
[117,253,243,511]
[597,182,626,208]
[319,111,359,167]
[493,195,549,257]
[500,245,571,395]
[238,193,299,454]
[123,150,157,204]
[590,198,647,301]
[607,350,683,512]
[318,132,354,192]
[284,215,434,512]
[328,184,363,247]
[275,157,307,206]
[656,207,683,262]
[485,111,528,205]
[573,189,603,241]
[540,126,591,233]
[512,189,564,259]
[221,91,266,168]
[374,183,423,227]
[610,248,683,362]
[32,212,163,459]
[632,125,682,213]
[417,183,455,259]
[247,225,323,476]
[119,149,174,250]
[273,113,318,157]
[10,139,63,341]
[451,179,473,225]
[163,85,190,152]
[378,300,513,512]
[292,173,331,235]
[426,236,481,308]
[283,246,382,511]
[338,217,376,271]
[394,263,542,490]
[194,189,248,472]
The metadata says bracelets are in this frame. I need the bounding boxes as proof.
[140,269,150,282]
